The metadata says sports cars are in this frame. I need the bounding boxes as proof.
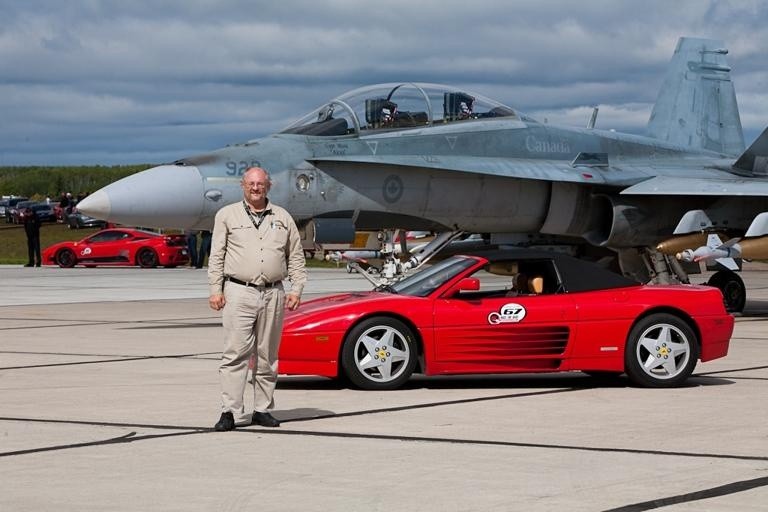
[248,248,735,390]
[40,228,189,269]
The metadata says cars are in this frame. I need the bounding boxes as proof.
[0,195,107,230]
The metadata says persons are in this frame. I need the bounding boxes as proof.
[207,166,309,429]
[47,190,90,225]
[23,207,42,268]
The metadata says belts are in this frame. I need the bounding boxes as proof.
[224,277,284,289]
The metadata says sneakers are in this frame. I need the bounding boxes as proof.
[23,262,42,268]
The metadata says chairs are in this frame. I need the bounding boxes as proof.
[505,273,527,296]
[518,275,543,296]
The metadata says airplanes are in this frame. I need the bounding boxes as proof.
[75,37,768,314]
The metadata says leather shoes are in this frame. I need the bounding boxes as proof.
[250,410,280,427]
[213,410,236,431]
